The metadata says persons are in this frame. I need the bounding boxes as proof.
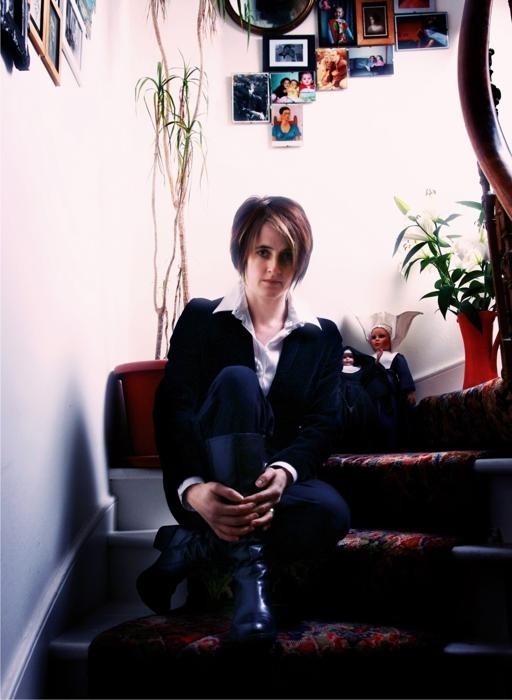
[132,195,352,649]
[316,55,333,90]
[277,79,300,103]
[272,105,302,141]
[270,76,291,102]
[365,13,384,34]
[276,43,293,62]
[324,50,347,88]
[286,49,302,61]
[370,54,386,72]
[366,322,418,453]
[352,56,376,74]
[326,6,355,46]
[342,343,390,454]
[238,83,267,119]
[295,70,314,98]
[416,16,437,49]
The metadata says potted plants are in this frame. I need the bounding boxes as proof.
[392,193,502,390]
[113,48,209,469]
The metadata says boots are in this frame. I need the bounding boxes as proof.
[135,525,277,643]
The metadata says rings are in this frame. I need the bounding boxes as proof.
[268,507,275,517]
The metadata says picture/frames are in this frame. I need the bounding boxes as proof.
[225,0,450,147]
[1,0,94,86]
